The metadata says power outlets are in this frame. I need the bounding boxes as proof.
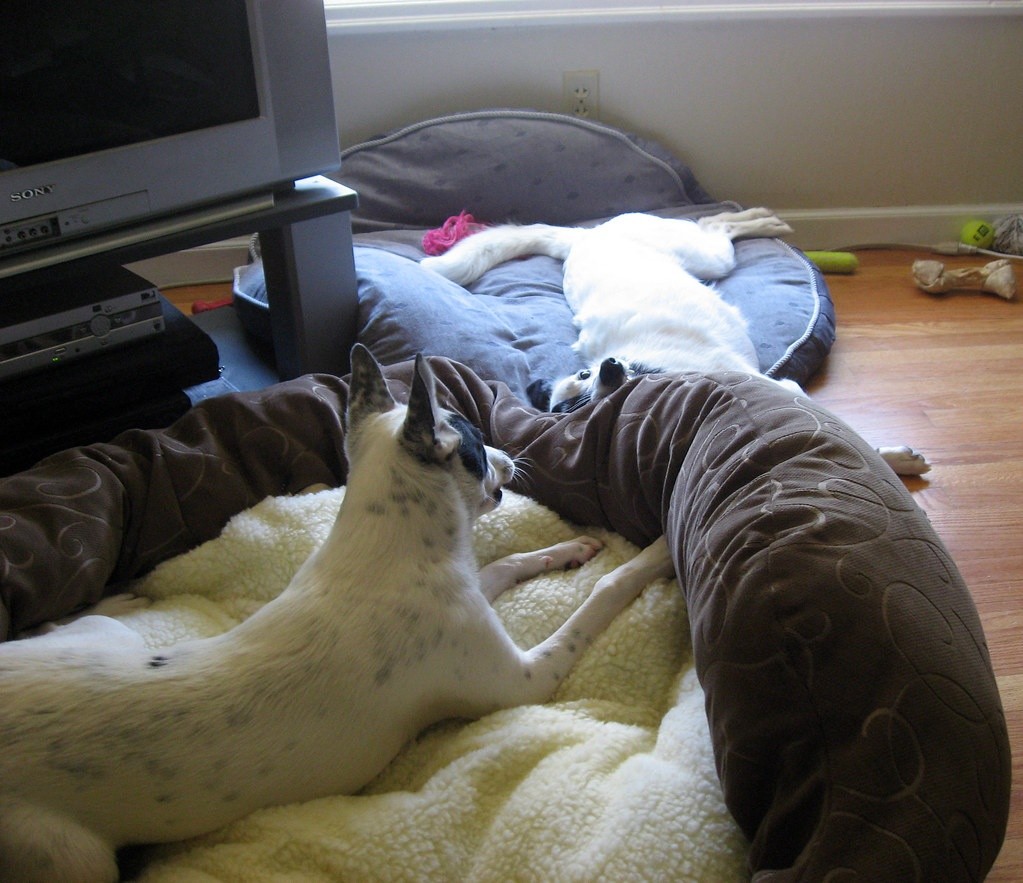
[562,69,599,120]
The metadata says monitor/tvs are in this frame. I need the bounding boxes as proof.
[0,0,342,259]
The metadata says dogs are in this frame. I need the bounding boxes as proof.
[0,344,682,883]
[418,205,935,477]
